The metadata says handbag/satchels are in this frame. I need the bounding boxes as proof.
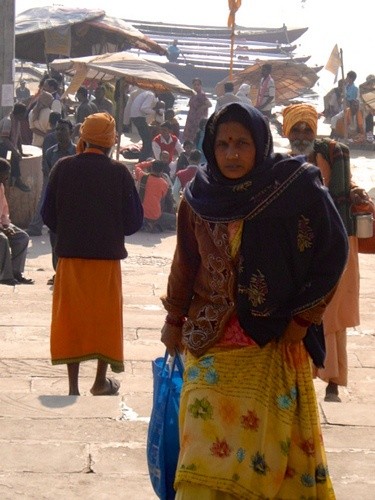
[146,343,185,500]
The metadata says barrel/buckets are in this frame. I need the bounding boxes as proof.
[348,199,374,238]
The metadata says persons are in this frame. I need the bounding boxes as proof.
[0,157,35,286]
[125,76,214,230]
[325,71,375,138]
[0,69,115,179]
[160,101,352,500]
[39,111,143,395]
[256,63,276,118]
[282,104,370,402]
[215,82,240,110]
[41,120,79,285]
[237,84,252,105]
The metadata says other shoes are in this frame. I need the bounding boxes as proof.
[0,274,35,285]
[324,394,342,402]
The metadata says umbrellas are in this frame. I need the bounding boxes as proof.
[215,58,319,106]
[54,50,197,161]
[12,5,169,90]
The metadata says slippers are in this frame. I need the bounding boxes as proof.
[96,376,120,395]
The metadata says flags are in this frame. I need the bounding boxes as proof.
[325,43,340,83]
